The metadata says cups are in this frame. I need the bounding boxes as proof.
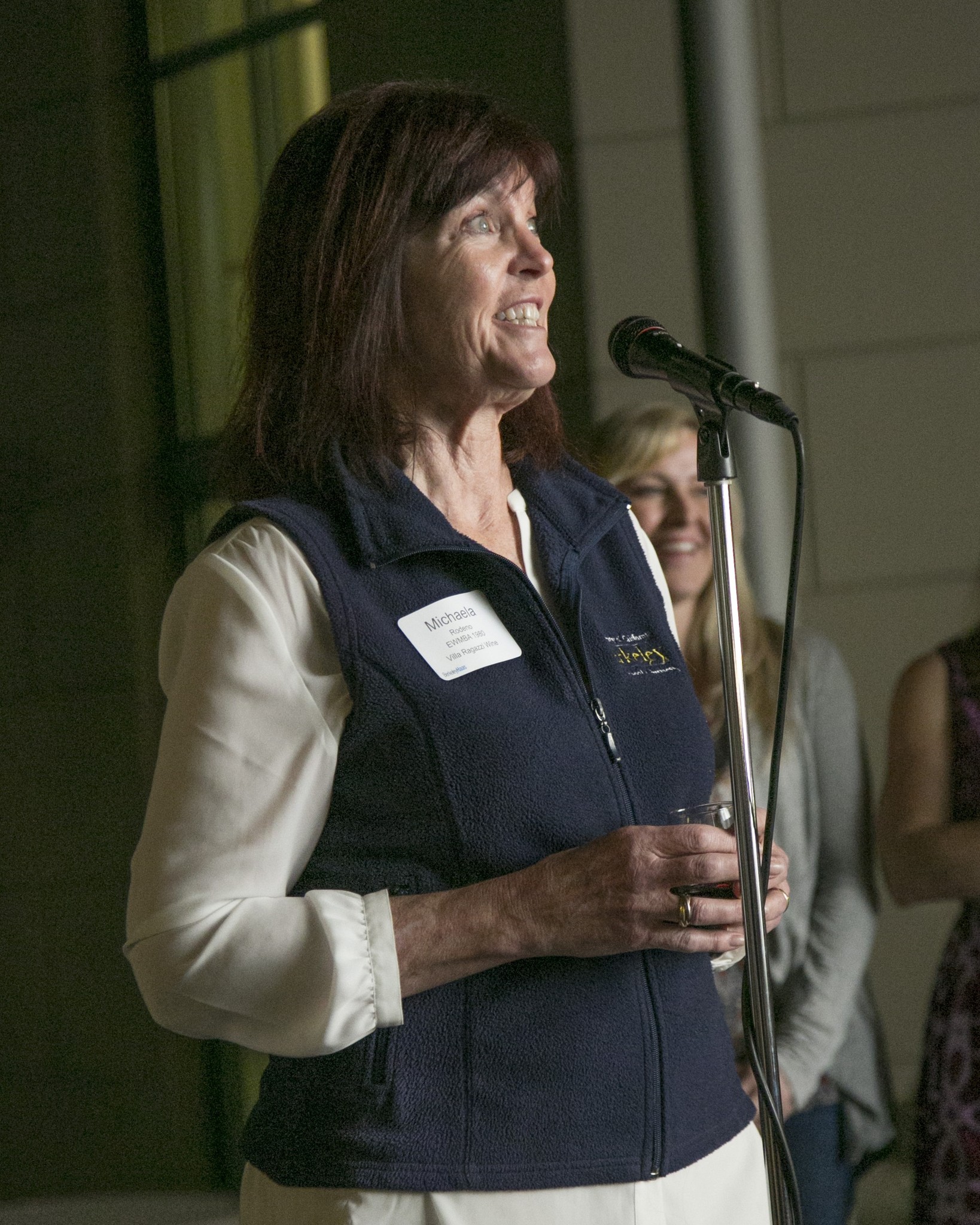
[668,801,740,932]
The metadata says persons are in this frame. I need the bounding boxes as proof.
[875,618,980,1222]
[122,88,784,1224]
[566,371,899,1224]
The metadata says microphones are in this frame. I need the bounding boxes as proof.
[607,314,802,431]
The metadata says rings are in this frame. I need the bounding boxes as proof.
[678,892,692,928]
[770,887,789,913]
